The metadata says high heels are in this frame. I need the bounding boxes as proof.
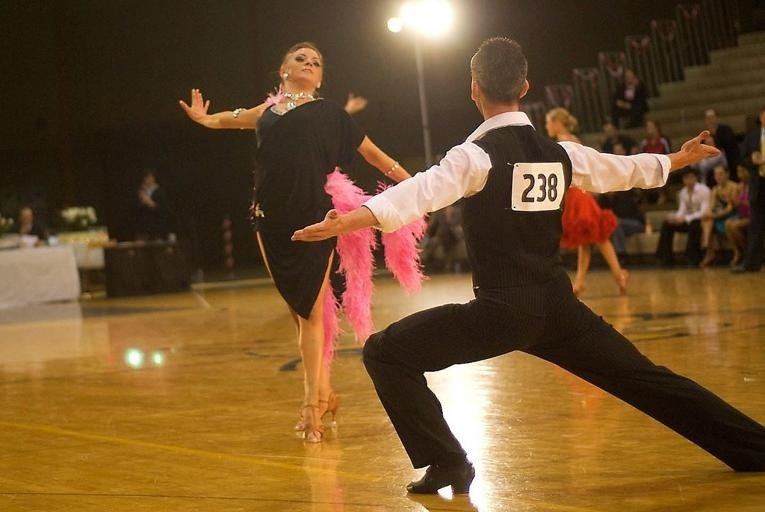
[295,390,339,444]
[407,461,476,495]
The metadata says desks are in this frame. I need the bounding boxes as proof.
[102,239,193,297]
[0,242,80,308]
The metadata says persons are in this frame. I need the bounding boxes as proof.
[134,170,169,239]
[8,207,49,247]
[418,204,467,275]
[180,42,429,443]
[290,38,764,494]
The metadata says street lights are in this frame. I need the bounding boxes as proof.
[401,0,459,248]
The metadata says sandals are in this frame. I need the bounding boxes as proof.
[571,281,586,294]
[617,268,629,291]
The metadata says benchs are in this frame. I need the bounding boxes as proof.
[624,203,729,252]
[581,33,763,146]
